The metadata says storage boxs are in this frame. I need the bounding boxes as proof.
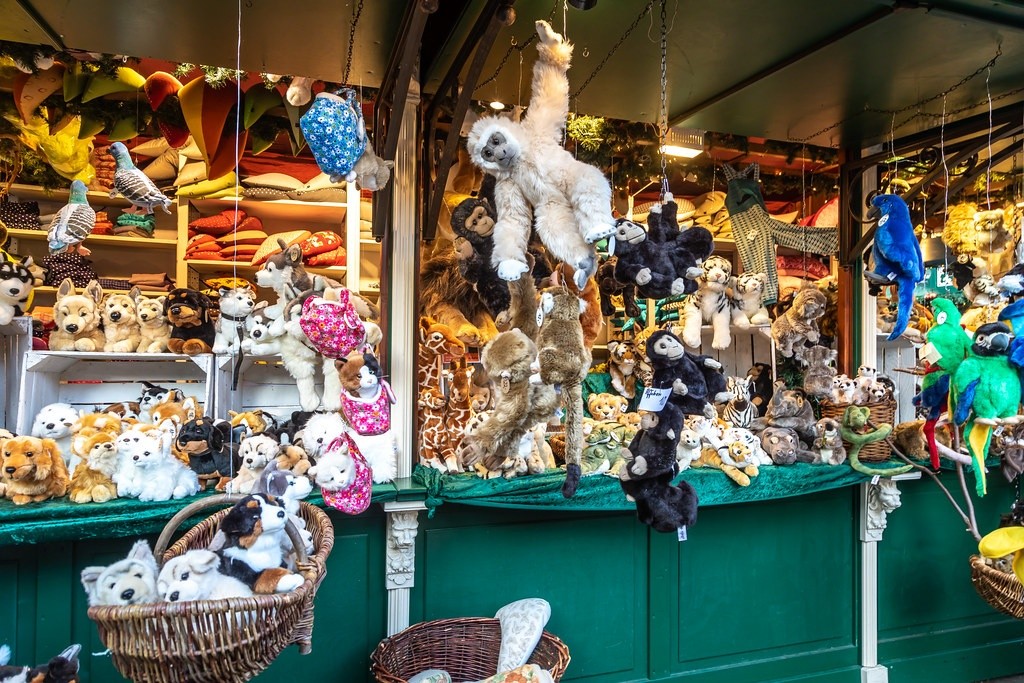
[671,323,777,383]
[214,346,342,421]
[13,346,214,444]
[0,315,36,435]
[878,331,922,428]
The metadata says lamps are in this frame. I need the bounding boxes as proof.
[658,127,705,157]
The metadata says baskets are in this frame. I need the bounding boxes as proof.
[88,492,334,683]
[969,554,1024,619]
[369,616,571,683]
[819,377,897,462]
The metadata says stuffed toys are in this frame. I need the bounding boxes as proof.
[156,549,265,631]
[908,203,1024,497]
[254,460,312,514]
[467,19,615,291]
[207,493,304,595]
[451,174,554,315]
[613,203,703,317]
[0,238,397,512]
[299,90,395,193]
[81,540,160,608]
[621,402,699,533]
[653,193,716,295]
[683,256,734,350]
[867,194,925,341]
[728,271,769,331]
[416,251,913,498]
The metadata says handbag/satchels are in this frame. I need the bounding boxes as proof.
[300,87,367,178]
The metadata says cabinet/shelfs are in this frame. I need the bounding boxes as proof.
[0,147,839,296]
